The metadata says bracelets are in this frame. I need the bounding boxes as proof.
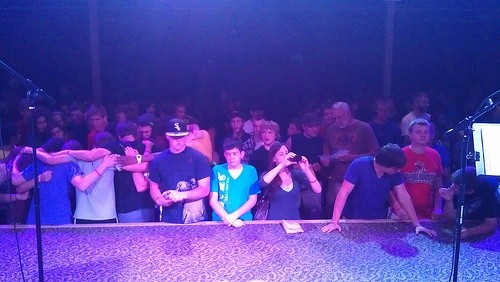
[67,151,70,154]
[14,194,17,200]
[95,170,102,177]
[414,224,420,229]
[310,178,316,183]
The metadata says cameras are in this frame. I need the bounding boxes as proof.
[293,155,302,162]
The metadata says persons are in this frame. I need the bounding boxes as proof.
[0,102,220,224]
[223,90,500,240]
[208,138,261,227]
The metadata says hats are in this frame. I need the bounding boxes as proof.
[302,113,320,124]
[375,143,406,169]
[165,118,190,137]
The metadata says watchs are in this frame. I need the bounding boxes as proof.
[137,154,142,163]
